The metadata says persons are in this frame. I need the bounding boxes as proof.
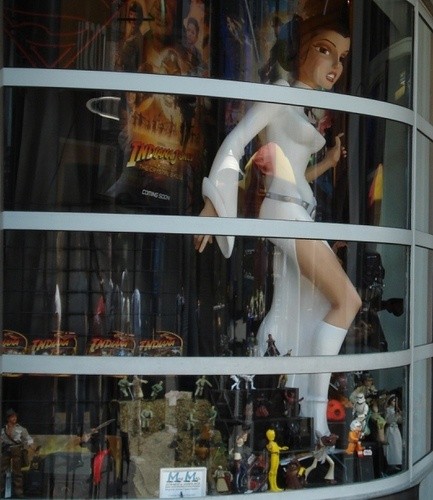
[78,427,110,487]
[191,13,365,447]
[0,407,35,498]
[214,372,403,494]
